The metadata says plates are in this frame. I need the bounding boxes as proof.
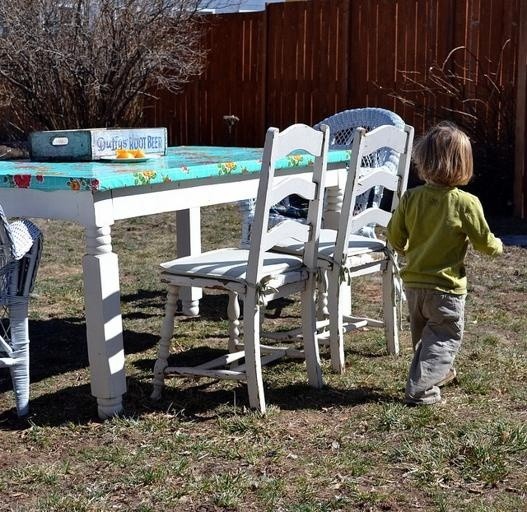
[99,154,160,162]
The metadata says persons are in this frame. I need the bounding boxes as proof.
[386,119,504,406]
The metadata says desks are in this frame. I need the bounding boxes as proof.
[0,144,354,417]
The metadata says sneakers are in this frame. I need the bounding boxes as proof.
[434,367,457,387]
[403,385,442,405]
